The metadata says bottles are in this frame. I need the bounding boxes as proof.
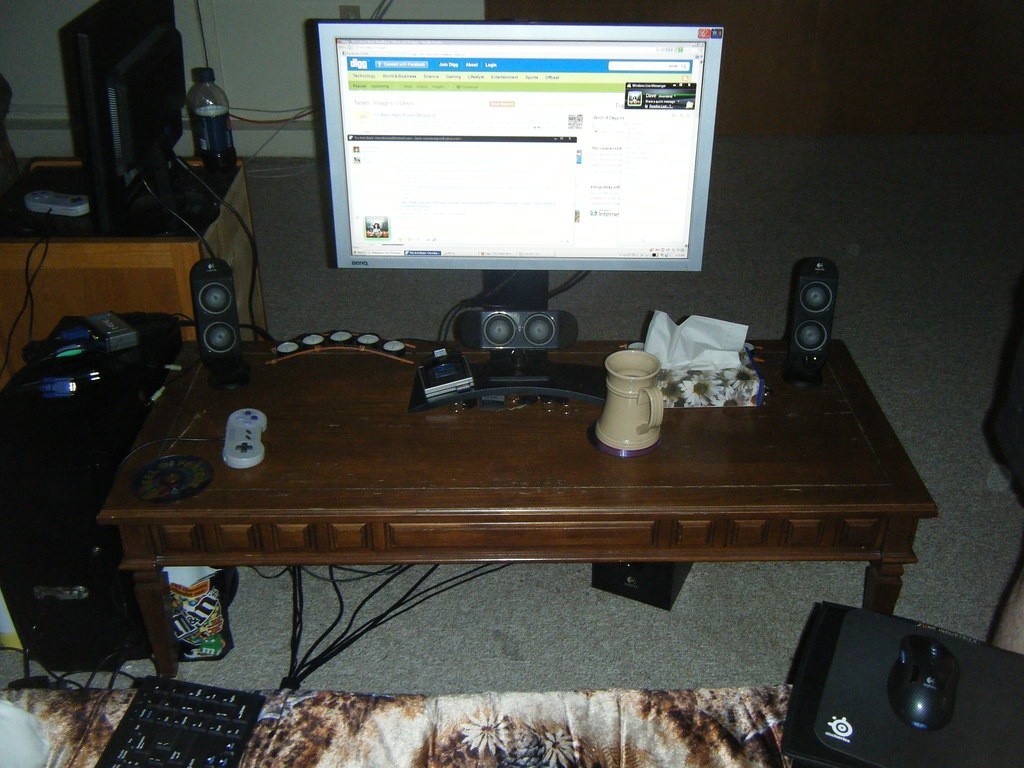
[191,67,236,172]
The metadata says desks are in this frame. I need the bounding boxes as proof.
[777,602,1024,768]
[98,342,937,679]
[0,157,266,389]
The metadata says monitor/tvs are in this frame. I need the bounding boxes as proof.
[307,18,726,415]
[78,0,204,235]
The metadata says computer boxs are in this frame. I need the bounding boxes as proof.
[0,345,238,672]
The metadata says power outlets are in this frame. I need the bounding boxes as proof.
[338,5,361,19]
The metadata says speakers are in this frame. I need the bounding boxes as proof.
[592,561,693,611]
[454,309,578,351]
[191,259,251,388]
[781,256,839,387]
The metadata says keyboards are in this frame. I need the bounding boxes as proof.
[94,676,267,768]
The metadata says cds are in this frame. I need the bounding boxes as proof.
[132,454,213,505]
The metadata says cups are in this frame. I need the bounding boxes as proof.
[593,350,664,456]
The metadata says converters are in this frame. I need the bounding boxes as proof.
[81,311,139,352]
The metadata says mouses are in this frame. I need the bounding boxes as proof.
[887,633,959,731]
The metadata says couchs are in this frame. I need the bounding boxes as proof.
[0,688,793,768]
